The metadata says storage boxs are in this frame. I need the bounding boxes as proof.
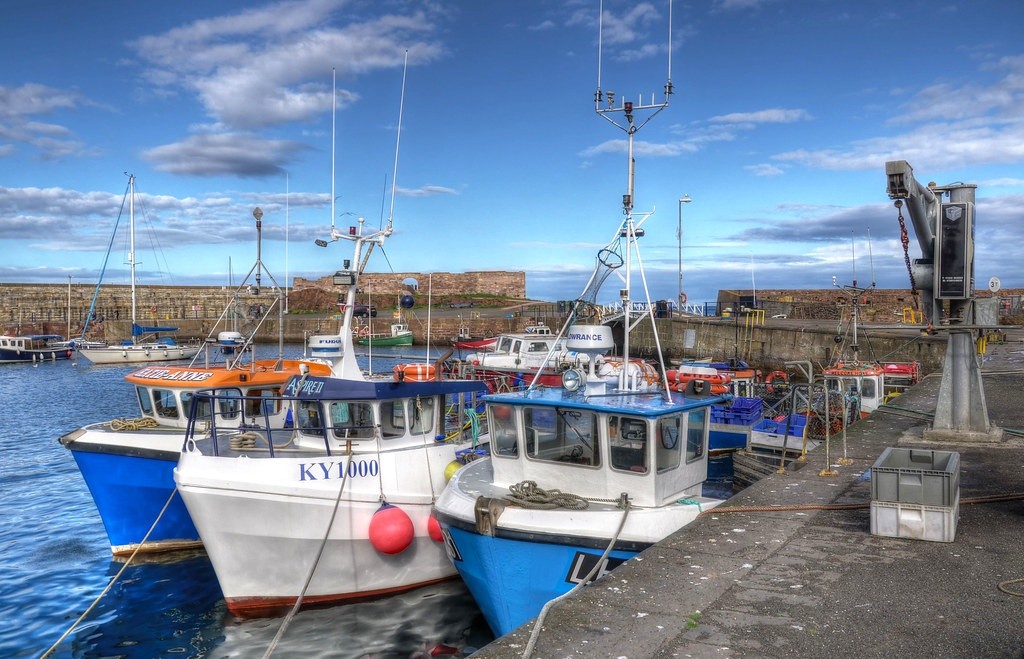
[870,447,961,543]
[453,390,489,413]
[711,396,764,425]
[752,414,807,437]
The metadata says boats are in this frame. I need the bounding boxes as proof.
[27,334,75,349]
[171,361,558,622]
[58,47,418,558]
[466,319,923,485]
[213,331,254,354]
[52,338,108,349]
[0,334,71,363]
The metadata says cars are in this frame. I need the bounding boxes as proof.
[354,303,378,318]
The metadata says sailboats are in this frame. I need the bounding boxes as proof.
[76,169,204,363]
[430,0,728,641]
[358,290,414,346]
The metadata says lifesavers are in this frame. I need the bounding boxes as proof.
[393,363,445,375]
[664,382,732,393]
[765,371,789,394]
[665,370,731,384]
[392,375,436,383]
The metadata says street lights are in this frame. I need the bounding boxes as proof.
[67,273,75,341]
[676,193,693,320]
[249,205,263,294]
